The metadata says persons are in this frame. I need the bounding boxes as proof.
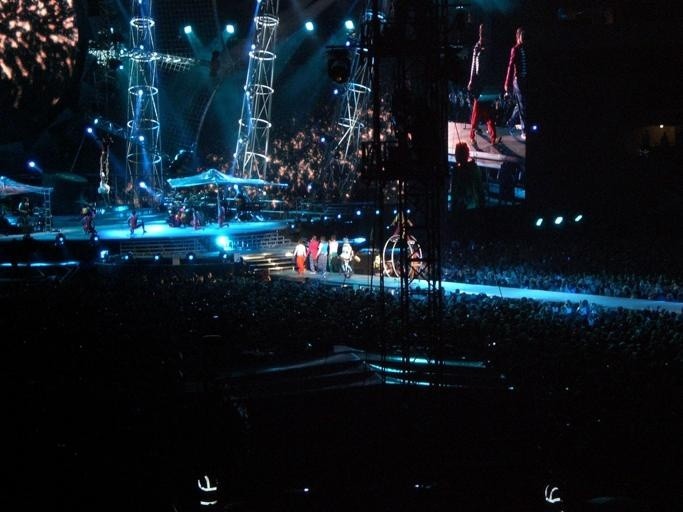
[467,24,500,143]
[505,24,526,142]
[2,99,683,512]
